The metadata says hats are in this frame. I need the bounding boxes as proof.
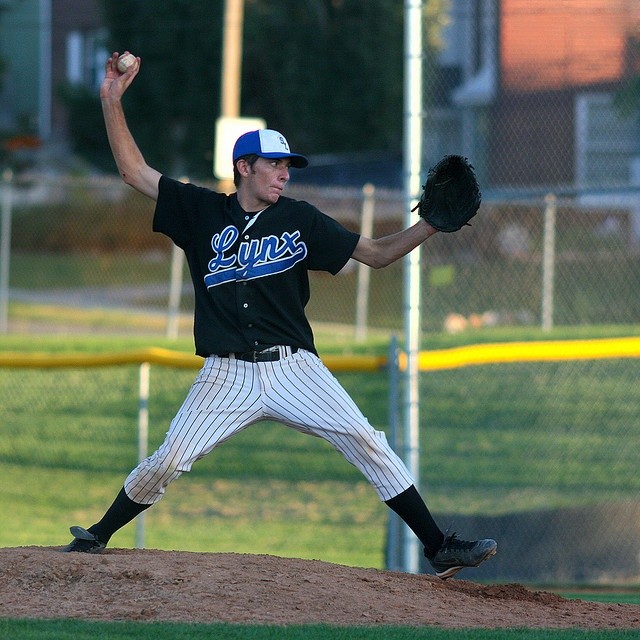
[233,129,308,169]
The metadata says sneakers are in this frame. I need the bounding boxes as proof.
[62,526,107,554]
[423,532,498,580]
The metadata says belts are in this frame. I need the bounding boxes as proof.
[207,345,297,363]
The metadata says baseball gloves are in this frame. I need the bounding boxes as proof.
[417,156,481,232]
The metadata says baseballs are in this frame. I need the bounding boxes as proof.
[116,52,135,72]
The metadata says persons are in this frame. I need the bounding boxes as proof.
[56,51,497,580]
